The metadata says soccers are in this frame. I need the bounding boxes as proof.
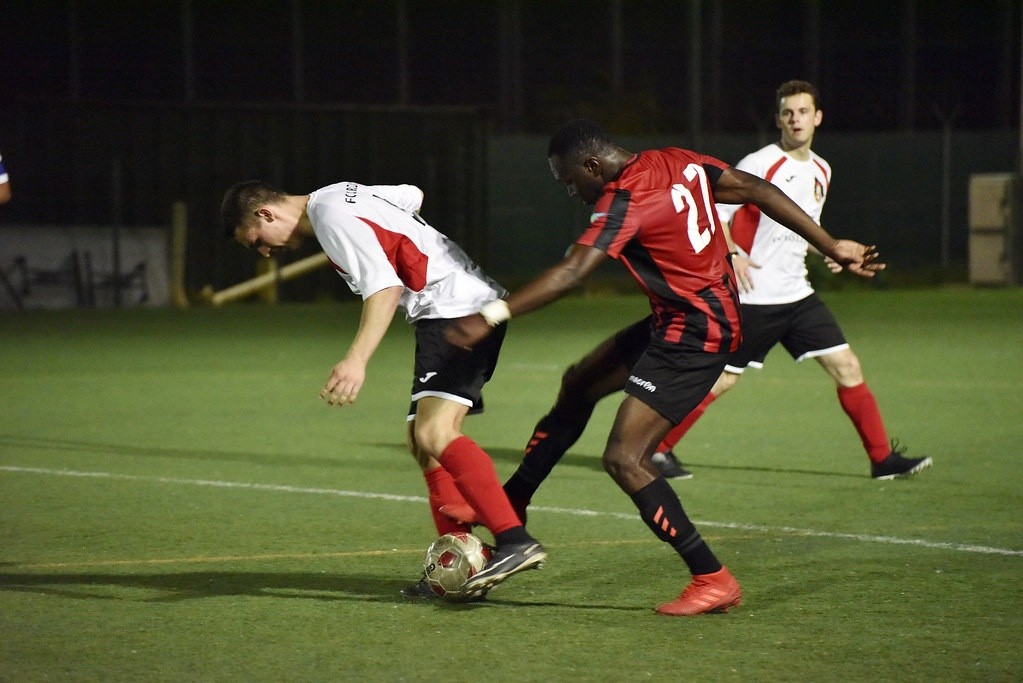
[423,530,493,603]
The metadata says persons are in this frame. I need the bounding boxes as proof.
[653,81,933,480]
[221,181,548,603]
[439,120,886,617]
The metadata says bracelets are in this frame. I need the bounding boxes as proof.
[731,252,738,257]
[480,299,512,327]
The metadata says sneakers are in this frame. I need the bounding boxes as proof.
[657,565,742,615]
[460,538,549,599]
[437,503,527,529]
[650,450,694,480]
[869,437,932,481]
